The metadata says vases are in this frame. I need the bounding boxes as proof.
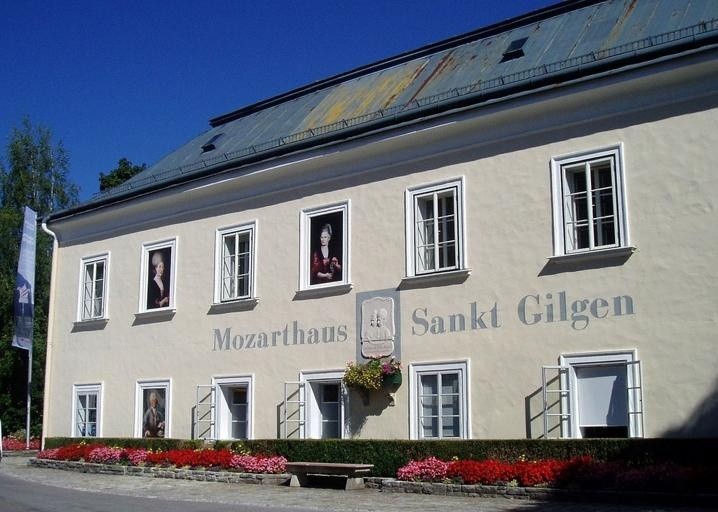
[384,374,402,384]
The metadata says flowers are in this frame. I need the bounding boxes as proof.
[383,356,402,376]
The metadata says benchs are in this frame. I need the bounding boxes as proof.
[284,462,375,490]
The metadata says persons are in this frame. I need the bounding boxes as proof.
[311,223,342,285]
[364,308,390,339]
[148,251,169,308]
[143,392,165,438]
